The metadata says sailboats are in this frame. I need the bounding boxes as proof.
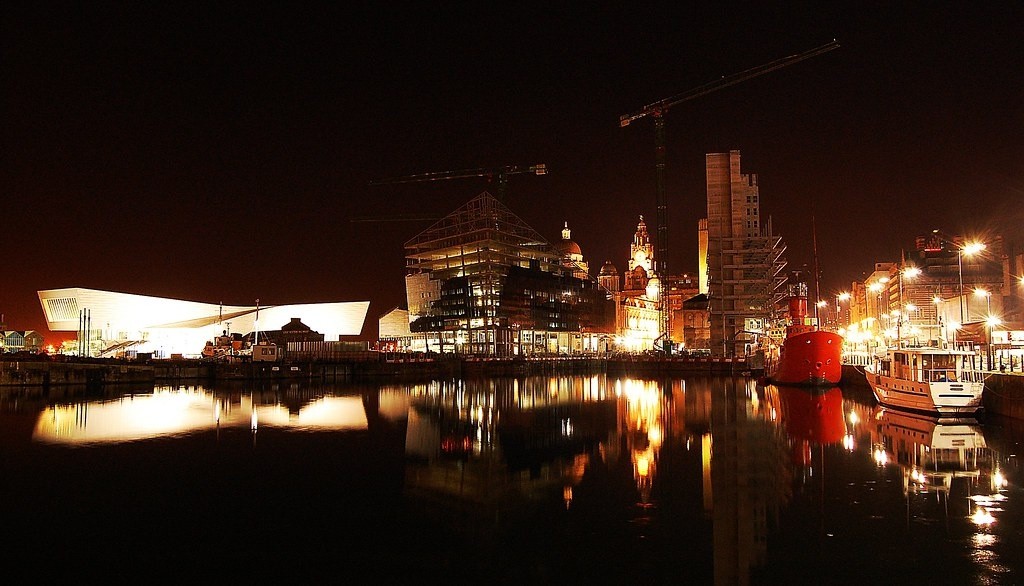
[775,213,842,389]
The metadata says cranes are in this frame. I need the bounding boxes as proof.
[368,164,547,203]
[619,40,841,354]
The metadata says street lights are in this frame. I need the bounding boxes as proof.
[958,244,985,326]
[865,284,884,351]
[849,297,944,349]
[898,269,922,349]
[816,292,851,346]
[979,291,992,321]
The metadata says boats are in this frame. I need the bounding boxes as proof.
[863,343,986,414]
